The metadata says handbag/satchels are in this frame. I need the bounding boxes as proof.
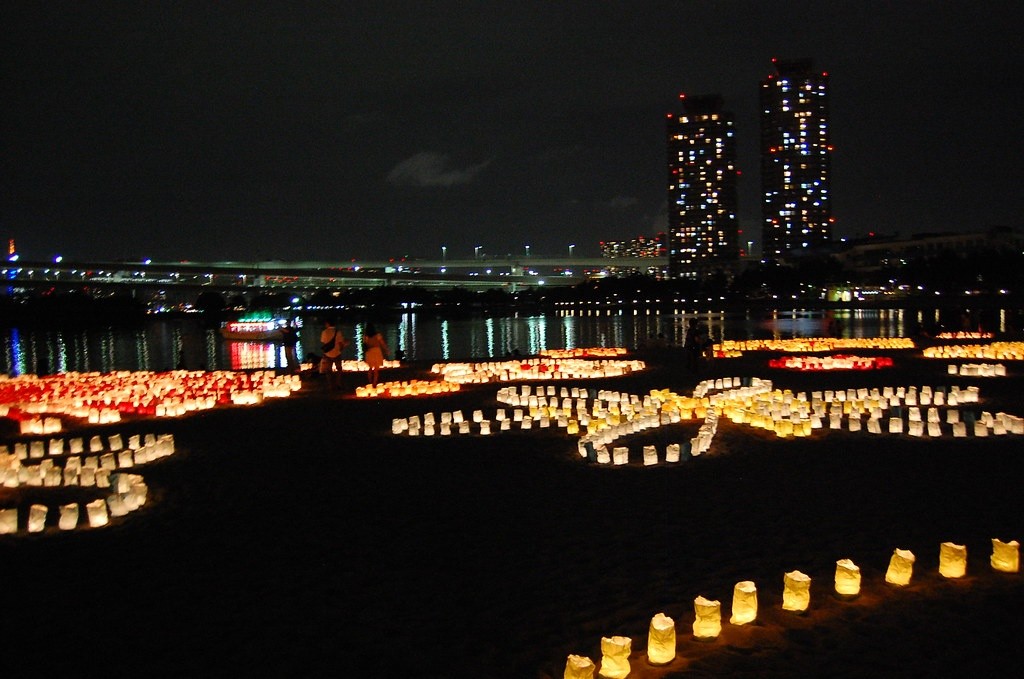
[321,330,337,353]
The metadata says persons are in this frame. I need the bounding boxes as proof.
[362,324,391,384]
[278,320,300,372]
[684,318,704,376]
[319,318,350,390]
